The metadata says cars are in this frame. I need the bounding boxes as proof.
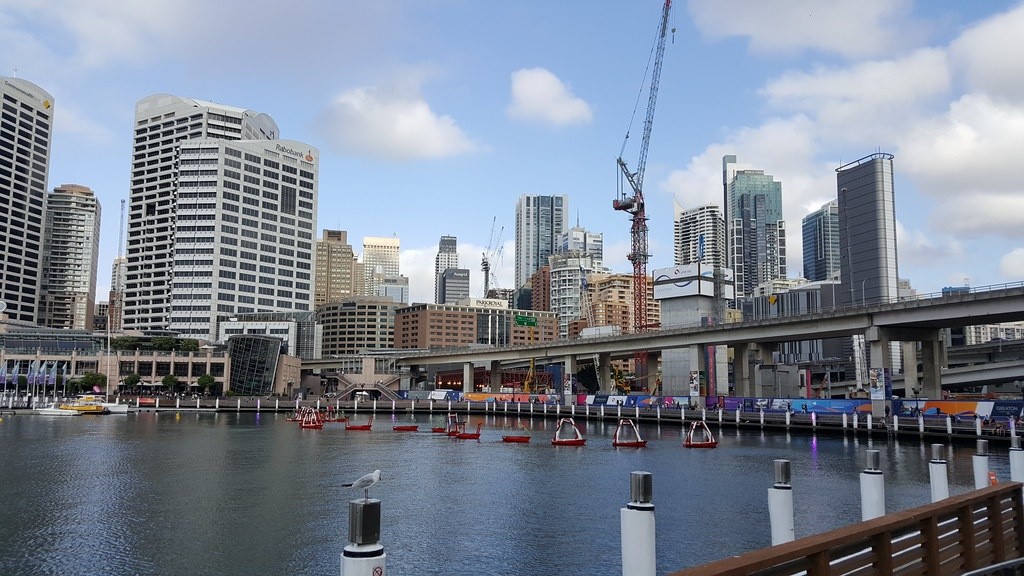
[984,336,1011,343]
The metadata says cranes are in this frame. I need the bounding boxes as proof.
[613,0,677,395]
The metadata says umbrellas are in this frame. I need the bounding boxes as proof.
[117,380,203,396]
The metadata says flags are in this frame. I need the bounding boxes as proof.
[0,360,67,386]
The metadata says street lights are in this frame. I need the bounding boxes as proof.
[863,278,871,308]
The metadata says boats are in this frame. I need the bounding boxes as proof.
[285,414,348,429]
[393,426,419,431]
[431,427,481,439]
[36,395,128,415]
[683,442,719,449]
[502,436,532,443]
[345,425,372,430]
[552,438,587,447]
[612,441,647,448]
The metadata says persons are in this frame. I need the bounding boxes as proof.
[117,388,211,402]
[266,396,270,402]
[494,396,520,406]
[416,394,420,403]
[885,406,890,422]
[800,402,808,416]
[876,417,885,431]
[784,403,795,417]
[617,398,726,415]
[528,396,545,405]
[950,413,1024,436]
[740,402,744,414]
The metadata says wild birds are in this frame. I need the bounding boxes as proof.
[341,470,381,502]
